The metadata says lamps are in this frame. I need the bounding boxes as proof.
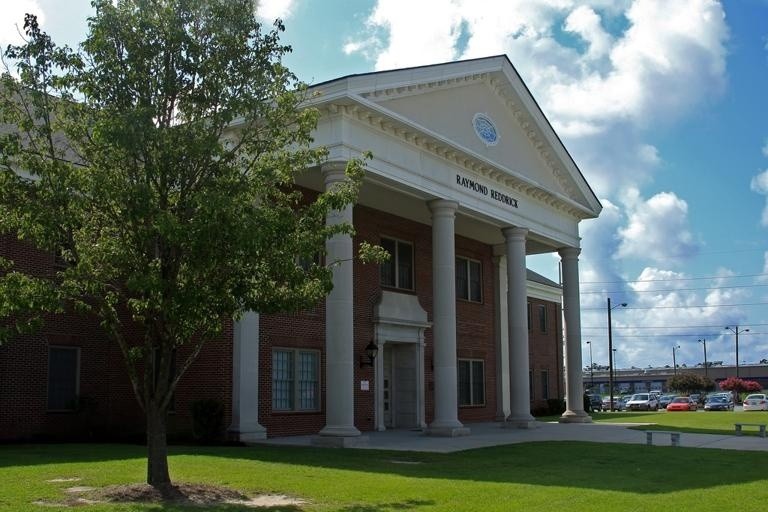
[359,340,378,367]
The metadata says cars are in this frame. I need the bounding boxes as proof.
[689,393,702,408]
[604,396,625,411]
[704,392,734,411]
[742,393,768,411]
[666,396,697,411]
[587,393,603,412]
[624,395,631,402]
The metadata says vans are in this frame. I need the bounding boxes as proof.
[651,391,673,407]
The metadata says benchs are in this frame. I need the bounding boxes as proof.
[734,422,767,438]
[645,431,681,448]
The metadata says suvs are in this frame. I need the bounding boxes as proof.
[626,393,658,411]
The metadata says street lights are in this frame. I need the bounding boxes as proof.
[698,338,707,377]
[585,341,593,389]
[606,298,628,412]
[725,326,749,379]
[672,345,680,376]
